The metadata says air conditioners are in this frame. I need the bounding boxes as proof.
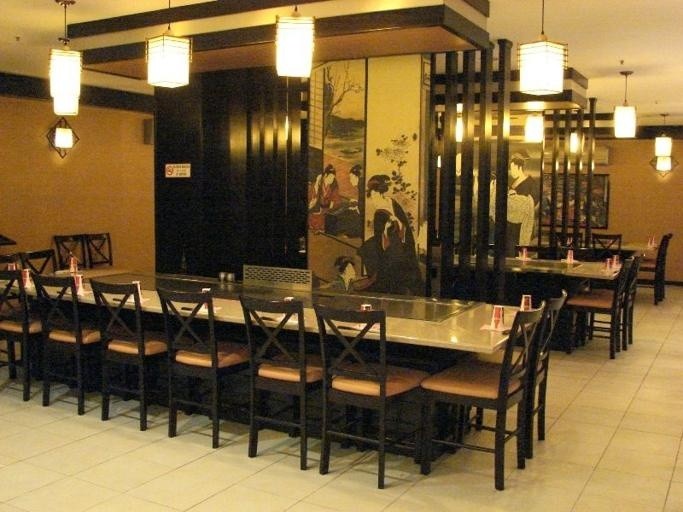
[542,145,609,165]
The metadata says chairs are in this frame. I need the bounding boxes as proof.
[592,233,622,250]
[420,301,546,490]
[89,277,168,431]
[30,270,102,416]
[313,303,432,490]
[566,256,634,359]
[1,247,58,275]
[553,232,583,249]
[466,290,568,459]
[155,287,250,449]
[639,231,674,304]
[1,270,43,402]
[53,231,113,271]
[588,256,640,351]
[238,294,353,471]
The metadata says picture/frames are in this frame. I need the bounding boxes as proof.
[541,173,611,230]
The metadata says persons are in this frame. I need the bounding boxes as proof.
[472,148,546,246]
[306,163,420,294]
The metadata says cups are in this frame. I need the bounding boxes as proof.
[72,275,84,292]
[19,268,32,289]
[201,287,211,308]
[69,256,80,273]
[8,263,15,272]
[218,272,235,286]
[605,258,613,273]
[283,296,295,322]
[521,295,531,311]
[361,304,372,312]
[612,255,620,267]
[519,248,528,258]
[492,305,503,327]
[131,281,141,299]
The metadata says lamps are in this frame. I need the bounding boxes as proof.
[649,114,679,178]
[515,0,569,98]
[46,0,82,158]
[613,70,637,140]
[143,0,194,90]
[274,0,317,79]
